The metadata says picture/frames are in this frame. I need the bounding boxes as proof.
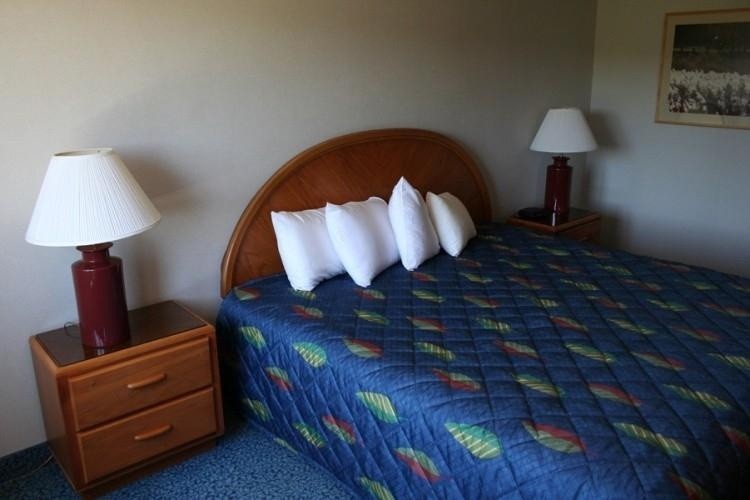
[653,7,749,131]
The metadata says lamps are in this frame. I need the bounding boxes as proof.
[25,145,162,346]
[530,108,599,214]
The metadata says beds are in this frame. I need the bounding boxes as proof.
[222,127,750,499]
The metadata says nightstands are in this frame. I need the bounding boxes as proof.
[28,296,226,500]
[507,207,603,240]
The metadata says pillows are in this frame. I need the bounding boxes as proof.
[327,198,402,285]
[271,207,349,293]
[388,177,442,270]
[426,187,478,257]
